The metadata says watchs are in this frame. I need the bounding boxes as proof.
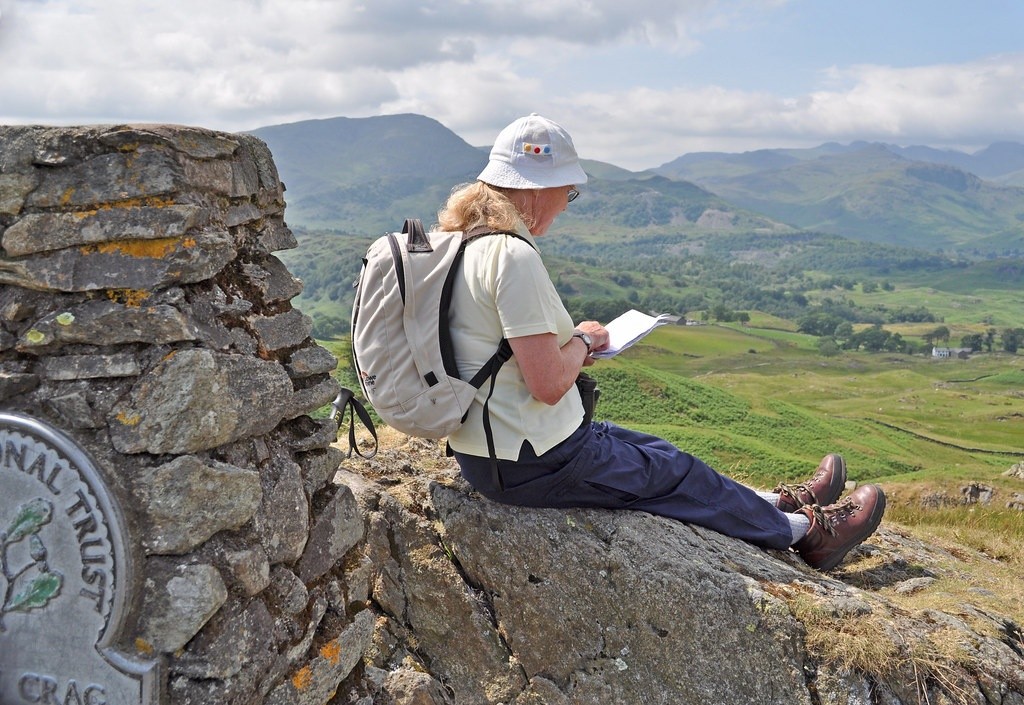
[573,333,593,358]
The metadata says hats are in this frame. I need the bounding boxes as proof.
[477,113,588,190]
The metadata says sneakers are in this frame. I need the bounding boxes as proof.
[772,453,848,513]
[792,484,886,572]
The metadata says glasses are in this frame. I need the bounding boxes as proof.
[568,185,579,202]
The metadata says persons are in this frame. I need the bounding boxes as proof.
[436,112,886,571]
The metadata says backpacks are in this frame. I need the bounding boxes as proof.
[351,219,535,439]
